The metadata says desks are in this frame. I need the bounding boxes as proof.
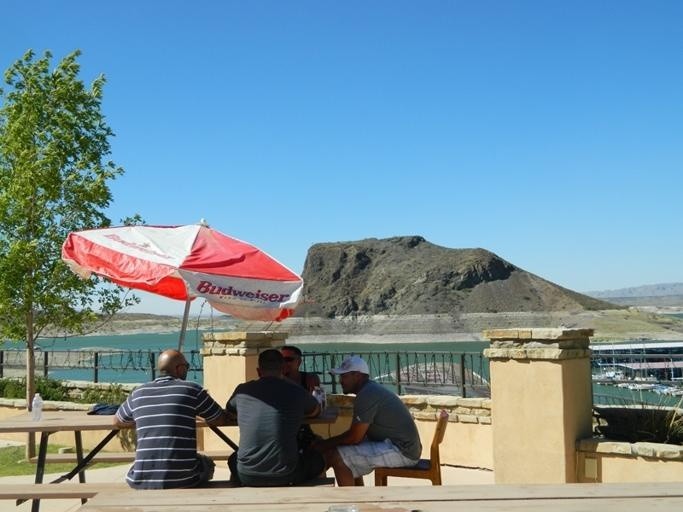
[0,406,340,511]
[76,481,682,512]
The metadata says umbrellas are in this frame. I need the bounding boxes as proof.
[61,218,305,353]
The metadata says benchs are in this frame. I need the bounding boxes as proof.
[1,480,334,511]
[15,449,239,504]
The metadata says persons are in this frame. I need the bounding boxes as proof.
[225,345,326,488]
[112,348,226,490]
[325,356,422,486]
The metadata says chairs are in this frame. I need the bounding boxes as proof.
[373,410,449,485]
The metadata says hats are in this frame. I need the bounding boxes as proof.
[330,356,371,376]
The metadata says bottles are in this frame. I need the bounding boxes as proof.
[32,393,43,423]
[312,385,326,417]
